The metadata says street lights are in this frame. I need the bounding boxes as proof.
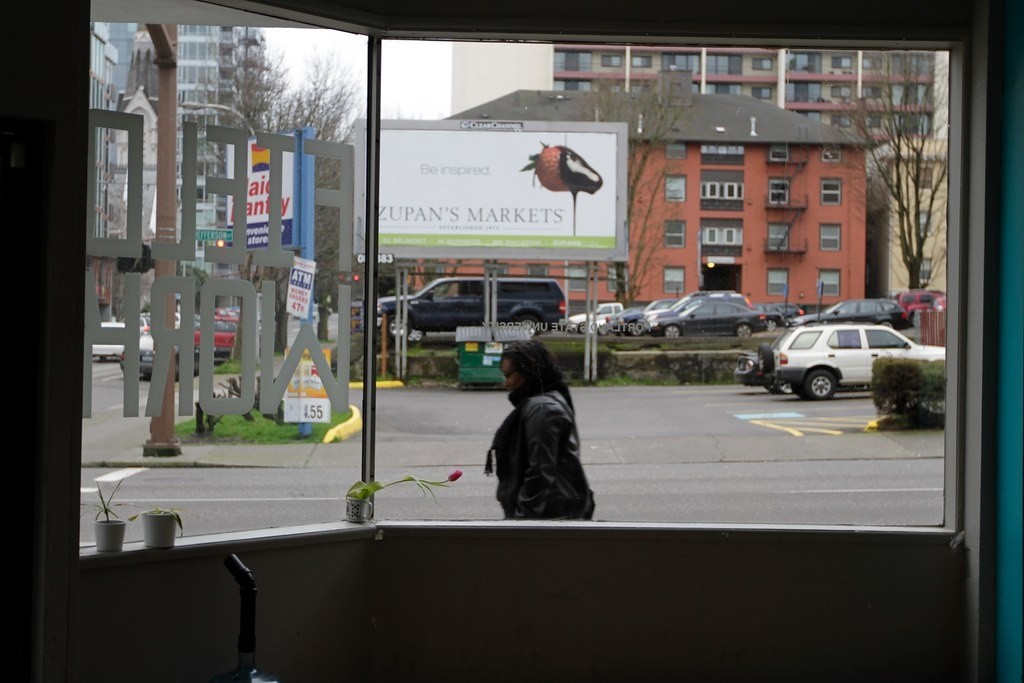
[181,100,254,137]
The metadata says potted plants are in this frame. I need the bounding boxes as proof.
[80,476,129,551]
[128,507,192,548]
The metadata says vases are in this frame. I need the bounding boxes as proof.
[345,497,373,523]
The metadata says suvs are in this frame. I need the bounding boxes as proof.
[789,298,907,330]
[757,322,946,400]
[377,276,567,341]
[194,315,240,358]
[893,290,946,328]
[644,290,754,335]
[754,302,784,332]
[777,303,804,322]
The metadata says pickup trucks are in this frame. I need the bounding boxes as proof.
[559,302,624,333]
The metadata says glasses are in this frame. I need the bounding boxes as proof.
[505,367,520,377]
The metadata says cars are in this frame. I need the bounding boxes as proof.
[606,298,679,339]
[126,311,181,333]
[734,327,793,395]
[120,329,199,381]
[646,301,767,338]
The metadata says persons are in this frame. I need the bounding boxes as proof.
[493,339,588,521]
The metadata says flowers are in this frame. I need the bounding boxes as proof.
[347,470,463,504]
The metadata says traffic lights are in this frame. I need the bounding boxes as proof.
[217,239,226,246]
[116,245,153,273]
[337,273,361,282]
